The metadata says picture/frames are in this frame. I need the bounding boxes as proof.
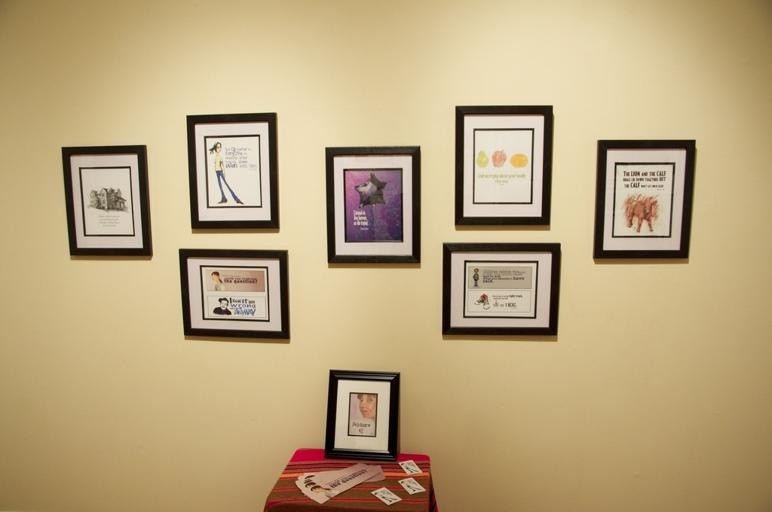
[186,111,280,229]
[323,145,425,264]
[454,106,552,228]
[324,366,401,467]
[437,240,562,335]
[59,145,152,258]
[175,247,289,335]
[592,138,696,258]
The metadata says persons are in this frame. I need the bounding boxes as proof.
[473,265,481,288]
[212,297,229,315]
[357,392,379,420]
[210,270,224,292]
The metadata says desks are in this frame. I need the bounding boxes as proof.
[260,448,437,511]
